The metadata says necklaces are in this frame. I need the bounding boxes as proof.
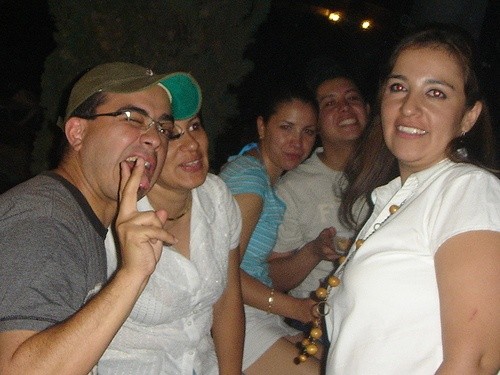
[165,193,194,224]
[291,161,447,365]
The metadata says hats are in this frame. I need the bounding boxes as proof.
[56,61,202,136]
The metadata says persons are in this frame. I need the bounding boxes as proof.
[298,27,500,375]
[218,82,327,375]
[90,61,246,375]
[268,63,389,325]
[0,64,203,375]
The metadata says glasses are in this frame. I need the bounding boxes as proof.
[77,110,185,140]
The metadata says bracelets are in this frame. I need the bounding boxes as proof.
[264,286,278,313]
